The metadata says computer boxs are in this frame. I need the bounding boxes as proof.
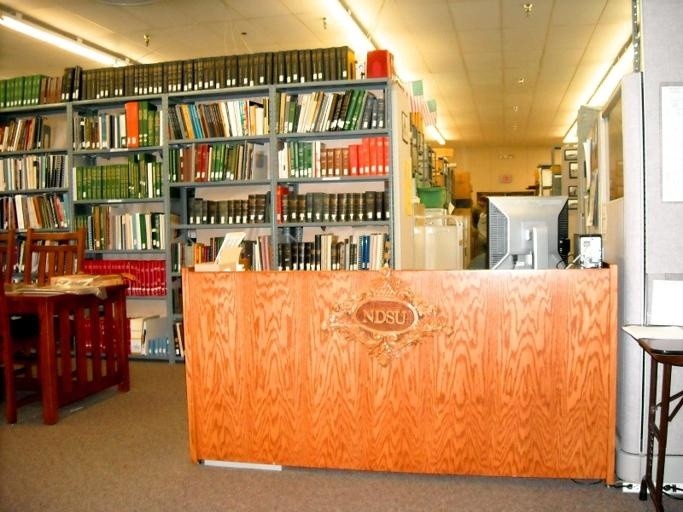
[574,233,603,267]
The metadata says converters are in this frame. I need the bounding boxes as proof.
[614,483,622,488]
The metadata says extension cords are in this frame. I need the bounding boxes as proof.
[622,483,683,494]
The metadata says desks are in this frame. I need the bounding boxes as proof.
[176,263,618,489]
[627,326,682,510]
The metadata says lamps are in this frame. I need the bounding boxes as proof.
[0,14,131,68]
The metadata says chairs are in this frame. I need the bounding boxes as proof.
[0,230,131,433]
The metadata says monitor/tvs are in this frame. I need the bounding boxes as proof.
[486,195,569,271]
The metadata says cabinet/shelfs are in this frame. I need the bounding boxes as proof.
[1,80,479,365]
[537,105,599,264]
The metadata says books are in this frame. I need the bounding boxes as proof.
[169,89,390,274]
[0,46,357,108]
[0,101,167,297]
[128,315,185,357]
